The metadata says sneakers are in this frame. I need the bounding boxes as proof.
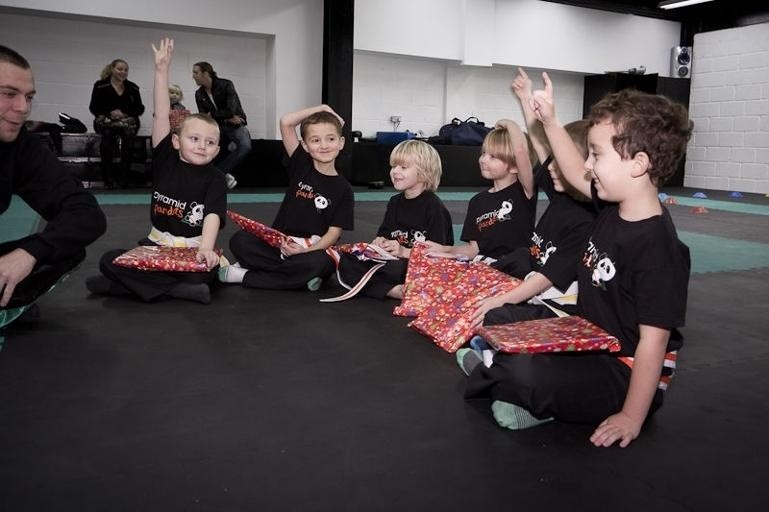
[226,173,237,189]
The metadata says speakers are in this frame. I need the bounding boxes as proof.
[671,46,693,78]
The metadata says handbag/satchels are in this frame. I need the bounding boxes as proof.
[93,116,136,135]
[439,117,492,145]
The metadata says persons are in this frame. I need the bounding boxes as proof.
[191,62,252,192]
[0,44,107,338]
[89,60,144,188]
[168,85,186,110]
[99,37,227,303]
[219,105,356,290]
[470,67,593,356]
[456,71,697,452]
[425,118,539,275]
[327,140,454,298]
